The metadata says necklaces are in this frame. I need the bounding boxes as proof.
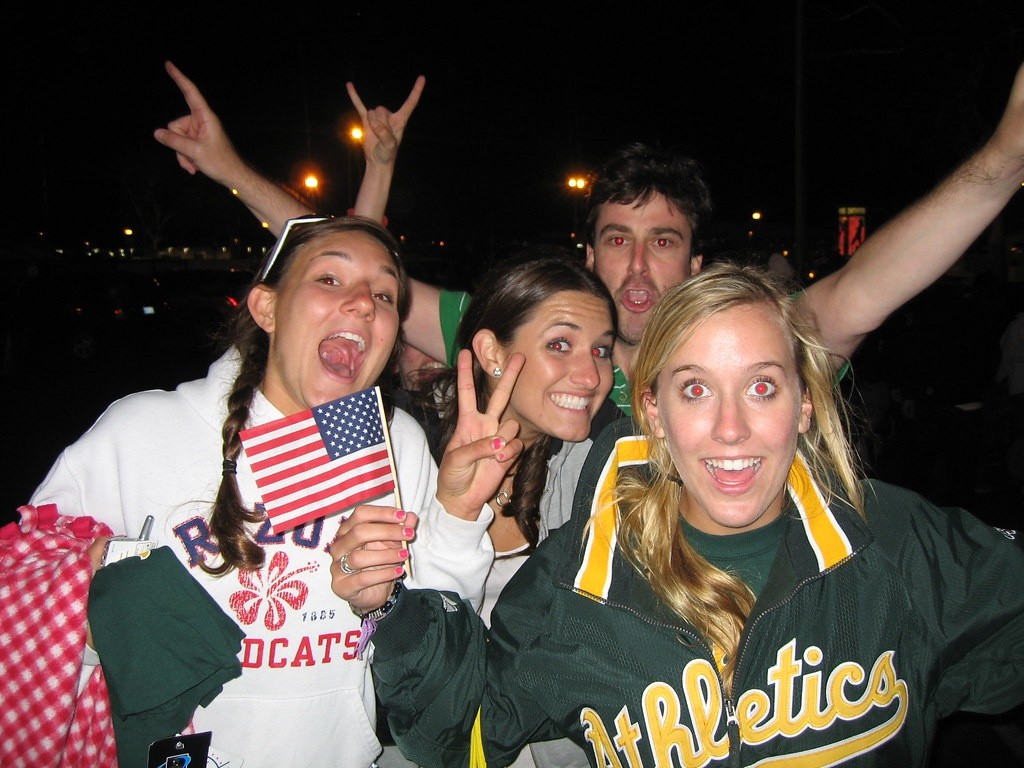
[496,476,514,507]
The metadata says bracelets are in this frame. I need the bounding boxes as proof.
[348,571,407,660]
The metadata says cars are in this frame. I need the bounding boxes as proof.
[1,256,244,366]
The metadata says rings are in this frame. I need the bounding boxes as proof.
[340,554,355,574]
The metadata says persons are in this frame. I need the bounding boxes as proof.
[19,212,528,768]
[398,256,621,768]
[152,60,1023,425]
[340,72,487,466]
[329,259,1024,768]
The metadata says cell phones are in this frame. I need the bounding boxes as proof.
[99,515,156,568]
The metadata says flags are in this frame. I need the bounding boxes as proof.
[238,388,395,535]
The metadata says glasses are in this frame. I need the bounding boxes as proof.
[260,214,404,282]
